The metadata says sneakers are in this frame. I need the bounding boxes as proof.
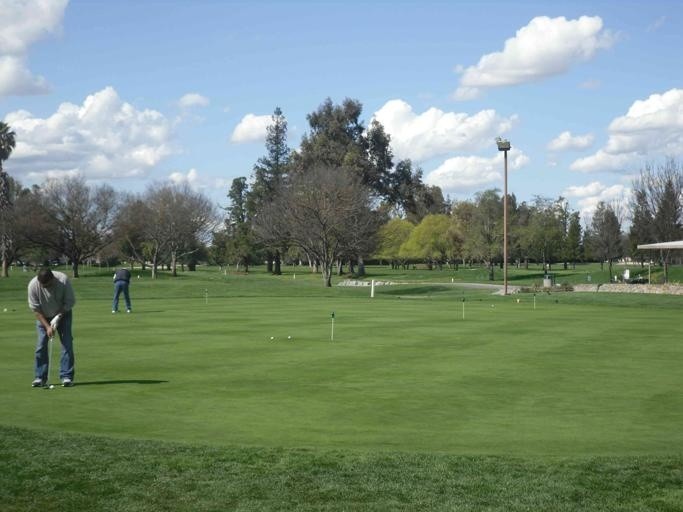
[111,311,116,313]
[126,309,130,313]
[31,379,46,386]
[62,379,72,386]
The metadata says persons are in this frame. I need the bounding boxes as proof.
[26,266,76,388]
[109,267,133,314]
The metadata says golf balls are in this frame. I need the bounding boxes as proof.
[49,385,54,389]
[4,308,7,311]
[491,304,495,308]
[271,336,275,340]
[288,336,291,339]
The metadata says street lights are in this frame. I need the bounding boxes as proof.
[499,142,510,296]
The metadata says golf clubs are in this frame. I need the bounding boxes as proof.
[43,336,52,389]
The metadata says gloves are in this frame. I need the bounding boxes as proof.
[49,313,62,330]
